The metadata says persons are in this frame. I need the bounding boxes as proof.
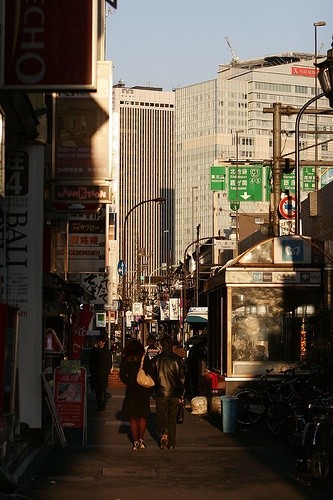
[102,331,122,362]
[125,331,203,410]
[89,335,112,411]
[151,336,186,450]
[119,336,150,451]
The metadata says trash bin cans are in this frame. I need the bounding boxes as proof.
[220,396,239,434]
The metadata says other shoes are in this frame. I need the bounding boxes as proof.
[160,428,170,449]
[97,407,105,411]
[168,444,175,450]
[132,441,146,452]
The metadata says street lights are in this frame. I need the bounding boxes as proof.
[120,196,165,356]
[148,263,181,305]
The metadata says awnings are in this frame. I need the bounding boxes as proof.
[184,307,209,322]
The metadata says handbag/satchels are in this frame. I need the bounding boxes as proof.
[137,355,155,388]
[177,397,184,424]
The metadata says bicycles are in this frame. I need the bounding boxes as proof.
[233,363,333,500]
